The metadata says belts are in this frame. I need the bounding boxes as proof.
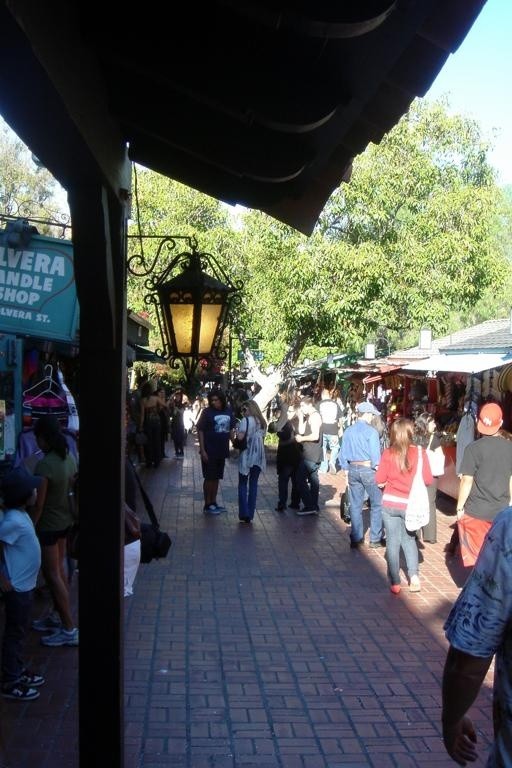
[349,461,370,465]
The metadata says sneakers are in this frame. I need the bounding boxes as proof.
[41,626,79,646]
[31,612,62,633]
[319,460,337,474]
[1,670,45,700]
[274,502,319,516]
[203,503,227,514]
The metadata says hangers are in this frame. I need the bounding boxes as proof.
[23,362,69,411]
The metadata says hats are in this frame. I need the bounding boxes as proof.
[1,465,47,497]
[357,401,382,416]
[477,403,503,436]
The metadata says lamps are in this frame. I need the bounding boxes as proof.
[127,233,244,370]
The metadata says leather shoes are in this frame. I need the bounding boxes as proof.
[410,577,421,592]
[390,584,400,594]
[351,537,364,549]
[370,539,386,548]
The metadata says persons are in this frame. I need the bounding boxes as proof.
[267,385,359,516]
[1,416,144,700]
[142,381,267,526]
[338,399,511,593]
[441,503,510,765]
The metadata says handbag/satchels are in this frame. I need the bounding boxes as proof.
[426,448,445,476]
[404,473,430,532]
[233,435,247,449]
[141,522,171,563]
